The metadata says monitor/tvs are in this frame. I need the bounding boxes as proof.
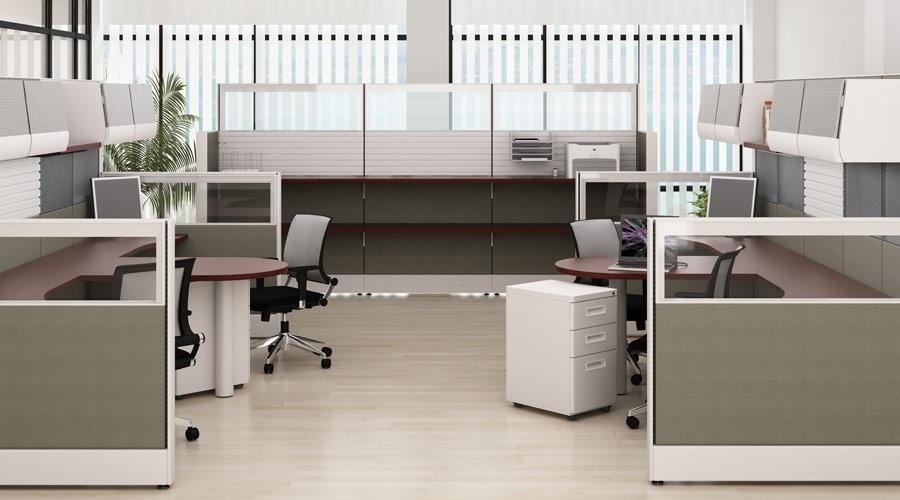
[706,176,758,218]
[91,176,141,219]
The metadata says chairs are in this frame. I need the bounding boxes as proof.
[250,213,337,374]
[626,242,746,429]
[570,218,649,385]
[111,258,202,442]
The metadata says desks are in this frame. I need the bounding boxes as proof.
[1,237,287,397]
[566,236,892,298]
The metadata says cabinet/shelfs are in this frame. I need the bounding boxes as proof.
[695,77,900,164]
[506,279,619,419]
[196,127,636,277]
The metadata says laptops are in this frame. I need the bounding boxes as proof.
[608,214,679,273]
